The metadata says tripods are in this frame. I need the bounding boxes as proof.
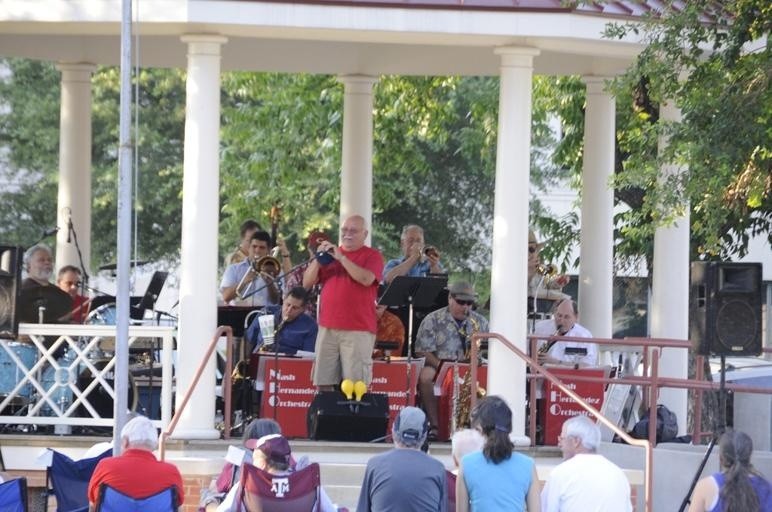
[0,310,59,433]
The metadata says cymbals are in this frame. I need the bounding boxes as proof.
[99,261,150,269]
[14,286,72,323]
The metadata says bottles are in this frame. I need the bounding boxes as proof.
[215,410,225,431]
[55,396,72,436]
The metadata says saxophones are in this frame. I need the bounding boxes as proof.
[449,310,485,441]
[231,316,289,385]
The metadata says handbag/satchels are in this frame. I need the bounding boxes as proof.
[634,404,678,440]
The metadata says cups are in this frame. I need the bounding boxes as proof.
[258,313,275,345]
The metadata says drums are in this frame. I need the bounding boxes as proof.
[84,303,122,352]
[1,340,39,406]
[41,359,138,436]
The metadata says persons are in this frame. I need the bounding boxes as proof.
[56,266,91,323]
[538,416,633,511]
[355,405,449,512]
[88,415,184,512]
[13,243,74,360]
[455,398,542,511]
[200,419,349,512]
[687,428,772,512]
[220,214,599,444]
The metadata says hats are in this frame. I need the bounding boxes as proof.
[479,396,512,431]
[391,407,429,441]
[245,434,291,464]
[448,279,476,301]
[529,231,546,250]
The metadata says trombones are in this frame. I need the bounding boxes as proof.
[235,246,281,299]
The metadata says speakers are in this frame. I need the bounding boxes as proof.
[0,245,23,340]
[307,390,389,443]
[691,261,762,356]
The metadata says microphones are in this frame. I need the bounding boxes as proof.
[319,245,327,257]
[74,279,93,293]
[67,210,71,242]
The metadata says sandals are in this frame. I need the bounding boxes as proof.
[427,425,439,442]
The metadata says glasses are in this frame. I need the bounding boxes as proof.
[530,247,536,254]
[450,293,473,306]
